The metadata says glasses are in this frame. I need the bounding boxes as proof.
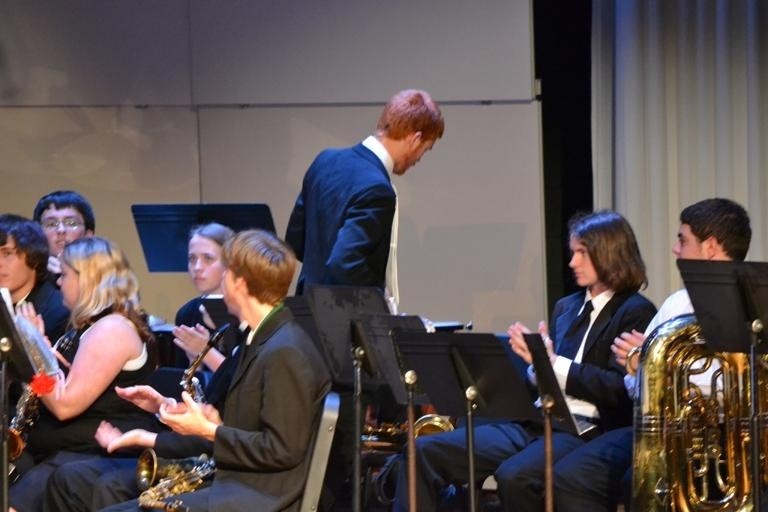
[42,218,87,231]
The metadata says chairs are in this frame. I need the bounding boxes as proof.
[296,391,343,512]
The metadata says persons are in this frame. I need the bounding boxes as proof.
[175,222,242,373]
[286,89,444,295]
[0,236,157,511]
[34,271,251,511]
[392,210,658,512]
[554,198,752,512]
[34,191,95,289]
[1,213,71,476]
[94,229,332,510]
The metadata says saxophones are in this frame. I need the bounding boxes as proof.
[138,447,216,512]
[366,414,454,444]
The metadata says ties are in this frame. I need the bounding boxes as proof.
[384,181,401,304]
[556,301,593,360]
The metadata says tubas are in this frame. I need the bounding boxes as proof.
[623,313,768,512]
